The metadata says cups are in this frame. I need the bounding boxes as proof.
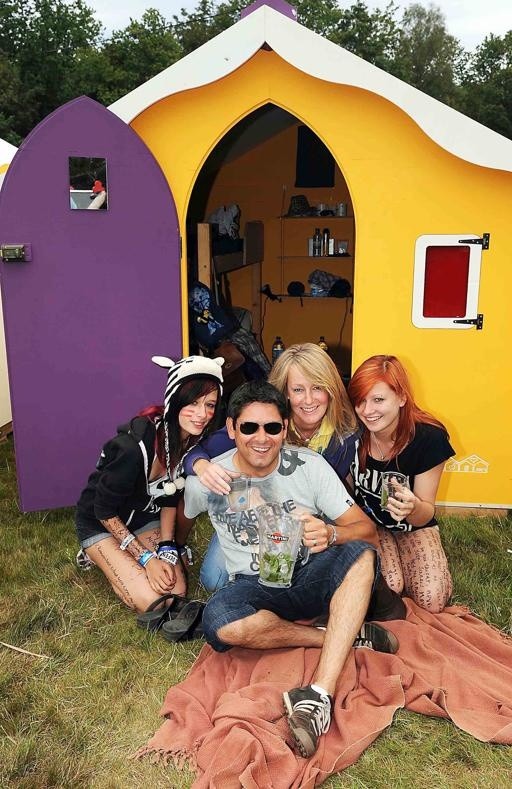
[381,472,406,511]
[317,203,328,216]
[226,477,249,512]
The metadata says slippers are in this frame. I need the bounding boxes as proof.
[162,597,208,643]
[136,593,191,629]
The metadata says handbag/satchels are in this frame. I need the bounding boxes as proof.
[210,343,245,376]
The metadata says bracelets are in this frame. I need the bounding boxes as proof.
[120,534,135,551]
[328,524,337,545]
[137,549,155,566]
[155,540,180,565]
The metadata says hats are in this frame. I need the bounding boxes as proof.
[151,355,226,404]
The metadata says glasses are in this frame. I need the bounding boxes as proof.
[240,422,283,435]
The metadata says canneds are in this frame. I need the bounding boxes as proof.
[317,203,326,215]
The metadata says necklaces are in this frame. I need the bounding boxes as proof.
[373,432,396,460]
[295,425,320,441]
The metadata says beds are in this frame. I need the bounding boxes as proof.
[184,221,264,384]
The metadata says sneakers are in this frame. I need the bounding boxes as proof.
[311,619,399,655]
[283,685,334,759]
[76,549,96,571]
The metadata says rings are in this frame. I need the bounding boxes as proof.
[312,539,317,546]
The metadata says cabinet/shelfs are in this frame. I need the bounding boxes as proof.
[277,215,355,306]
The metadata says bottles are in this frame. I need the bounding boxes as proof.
[317,335,328,353]
[313,228,330,257]
[271,336,285,365]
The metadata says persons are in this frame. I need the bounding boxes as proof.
[346,355,456,614]
[173,379,400,758]
[182,342,358,497]
[74,354,224,614]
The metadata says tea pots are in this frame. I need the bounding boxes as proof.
[252,502,314,589]
